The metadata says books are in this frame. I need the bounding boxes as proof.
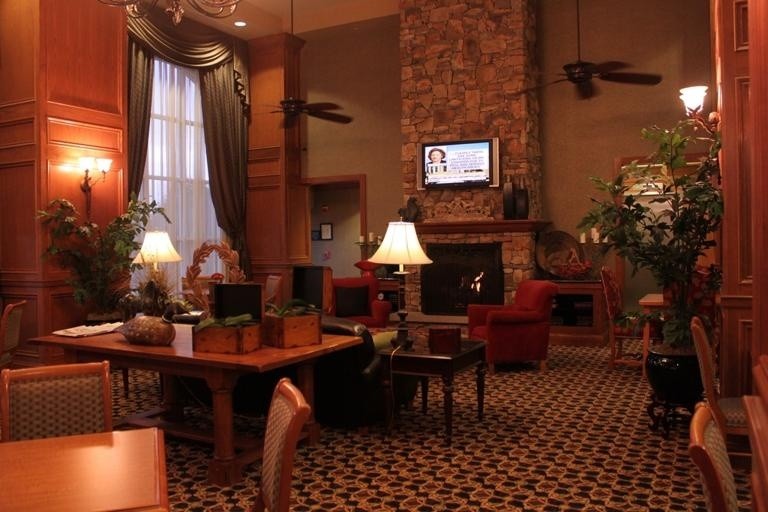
[51,320,124,338]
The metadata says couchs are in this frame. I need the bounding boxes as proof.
[467,279,561,375]
[334,276,386,326]
[173,306,419,430]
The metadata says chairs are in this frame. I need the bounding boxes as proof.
[1,300,28,368]
[250,378,311,512]
[0,360,112,444]
[599,266,663,373]
[690,317,768,474]
[689,400,768,511]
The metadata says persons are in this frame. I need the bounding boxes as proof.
[426,147,448,174]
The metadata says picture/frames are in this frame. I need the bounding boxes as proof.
[320,222,333,241]
[311,230,321,241]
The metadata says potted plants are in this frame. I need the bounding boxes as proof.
[192,316,263,356]
[263,301,325,349]
[36,192,172,327]
[576,119,726,440]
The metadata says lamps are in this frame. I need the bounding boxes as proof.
[56,155,113,191]
[133,229,182,280]
[679,84,718,136]
[369,221,434,348]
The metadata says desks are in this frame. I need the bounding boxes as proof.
[378,334,487,444]
[0,430,168,512]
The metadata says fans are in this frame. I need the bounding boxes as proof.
[254,1,354,129]
[506,0,664,101]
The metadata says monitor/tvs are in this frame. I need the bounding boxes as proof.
[416,136,502,190]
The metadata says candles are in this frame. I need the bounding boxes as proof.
[593,232,600,244]
[360,235,364,243]
[592,225,597,240]
[377,234,382,246]
[369,232,374,243]
[580,232,585,244]
